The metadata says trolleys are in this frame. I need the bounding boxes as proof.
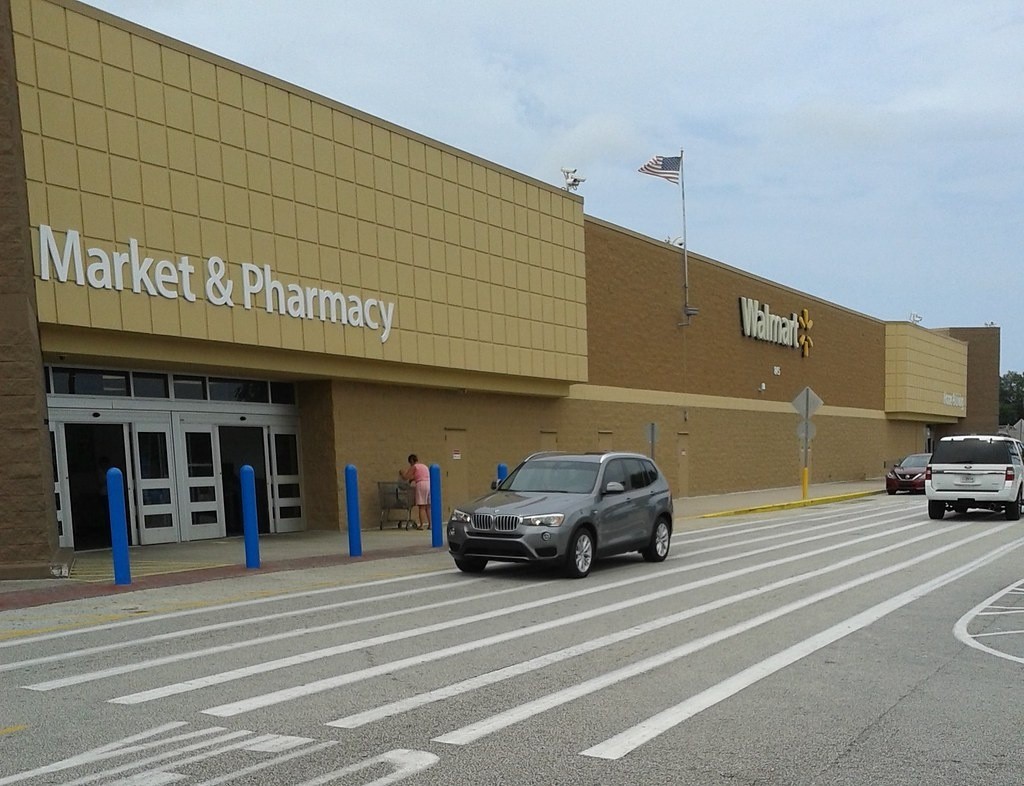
[375,482,418,531]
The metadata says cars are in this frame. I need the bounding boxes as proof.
[885,452,933,494]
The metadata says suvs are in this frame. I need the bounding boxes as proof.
[925,436,1024,518]
[448,447,675,577]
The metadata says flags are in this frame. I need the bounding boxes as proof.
[638,156,682,185]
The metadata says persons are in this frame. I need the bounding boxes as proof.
[399,454,431,530]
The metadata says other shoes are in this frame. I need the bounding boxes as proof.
[417,527,423,530]
[428,526,431,529]
